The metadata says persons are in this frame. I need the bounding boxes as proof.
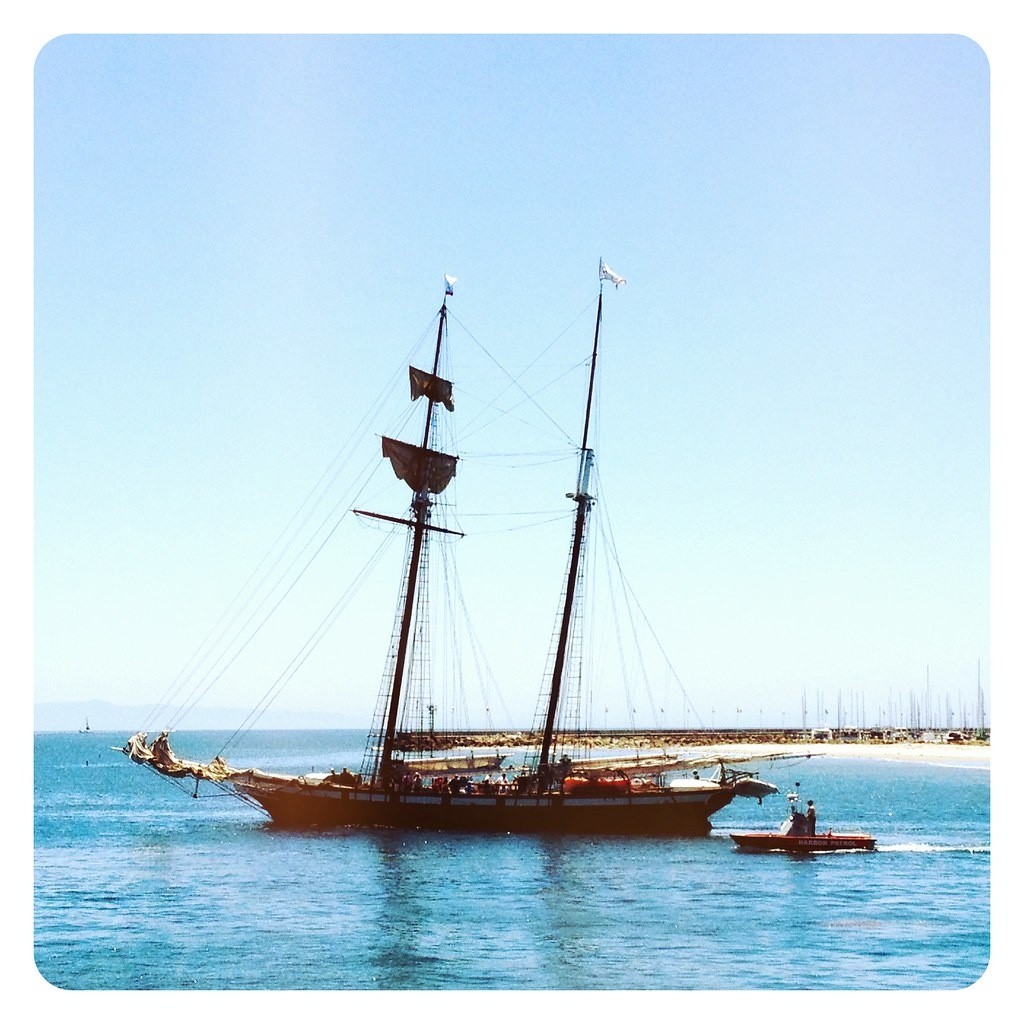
[692,770,700,780]
[806,798,817,833]
[401,769,535,795]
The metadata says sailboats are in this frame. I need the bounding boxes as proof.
[79,722,93,734]
[119,260,778,835]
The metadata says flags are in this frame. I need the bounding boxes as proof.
[596,256,627,290]
[445,274,456,295]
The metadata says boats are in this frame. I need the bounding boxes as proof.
[370,745,383,751]
[401,755,506,770]
[162,727,175,732]
[731,814,875,853]
